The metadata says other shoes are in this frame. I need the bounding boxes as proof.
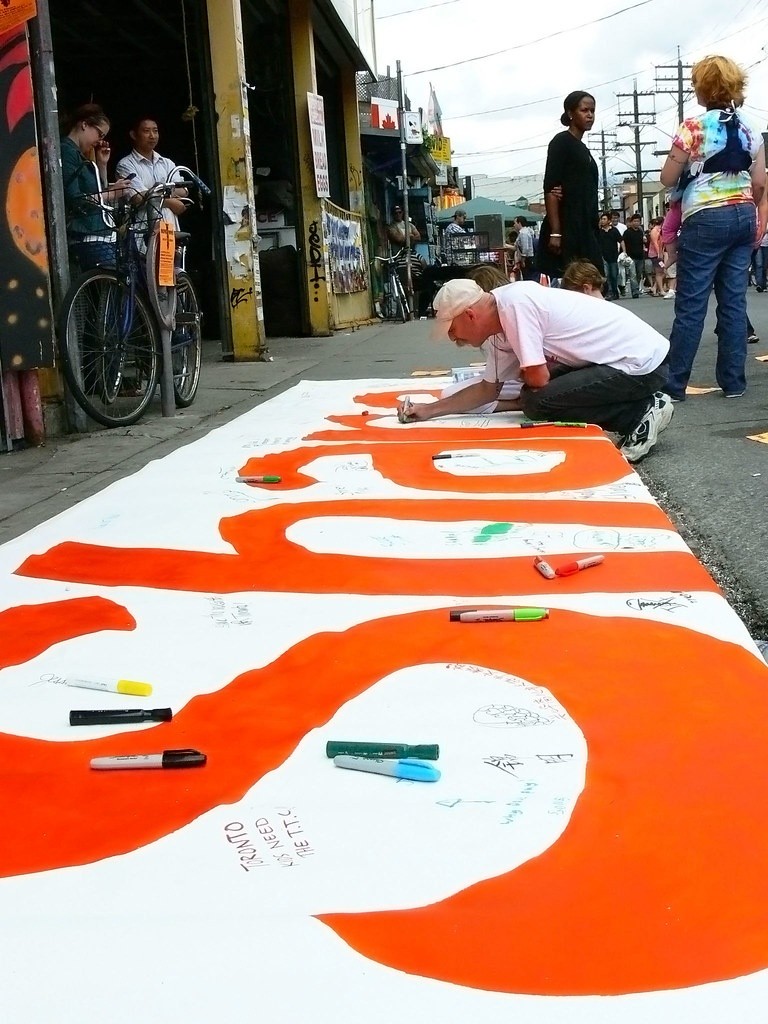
[640,287,677,299]
[604,288,627,301]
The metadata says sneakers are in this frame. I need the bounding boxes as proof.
[756,285,763,292]
[619,391,675,464]
[747,334,759,342]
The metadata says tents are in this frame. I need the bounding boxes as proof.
[436,196,543,228]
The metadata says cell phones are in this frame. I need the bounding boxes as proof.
[125,174,137,181]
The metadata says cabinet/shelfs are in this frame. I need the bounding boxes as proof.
[450,232,492,265]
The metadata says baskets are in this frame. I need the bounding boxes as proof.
[69,187,163,271]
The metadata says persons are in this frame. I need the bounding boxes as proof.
[539,91,605,279]
[445,211,468,266]
[655,56,767,402]
[397,262,675,462]
[387,205,434,317]
[505,210,768,300]
[60,104,188,396]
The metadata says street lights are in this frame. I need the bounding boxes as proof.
[599,155,644,228]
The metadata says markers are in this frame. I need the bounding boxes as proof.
[334,756,441,783]
[554,421,587,428]
[70,708,172,726]
[521,421,554,428]
[67,674,150,696]
[236,477,281,483]
[326,740,439,757]
[555,555,604,576]
[450,608,549,623]
[403,396,410,424]
[88,751,205,770]
[534,555,555,579]
[432,454,479,460]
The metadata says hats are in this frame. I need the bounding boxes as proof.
[451,209,467,217]
[390,204,403,210]
[433,278,483,340]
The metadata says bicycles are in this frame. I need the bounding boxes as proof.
[58,159,210,429]
[369,246,414,324]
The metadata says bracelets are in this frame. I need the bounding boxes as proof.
[550,232,562,237]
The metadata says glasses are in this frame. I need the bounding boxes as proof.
[92,123,106,140]
